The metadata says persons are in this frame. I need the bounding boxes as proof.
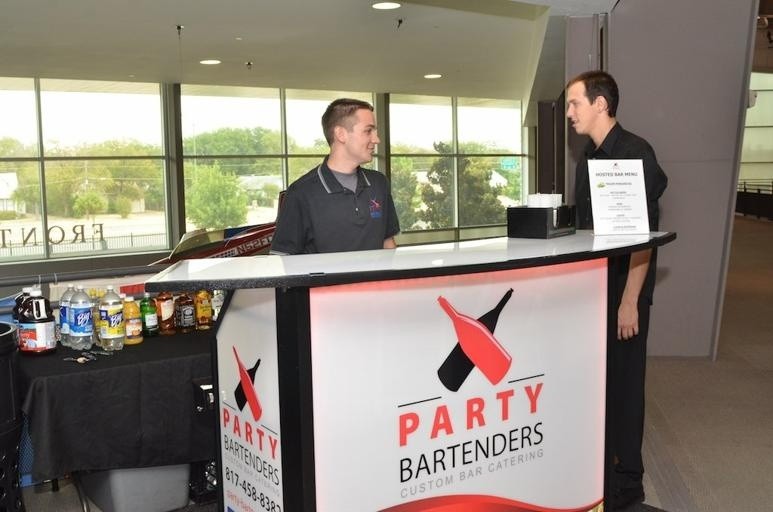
[563,71,670,512]
[268,97,400,256]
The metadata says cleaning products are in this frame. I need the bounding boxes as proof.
[124,290,213,346]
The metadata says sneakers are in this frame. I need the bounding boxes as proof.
[605,485,645,511]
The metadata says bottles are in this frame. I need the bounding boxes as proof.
[11,283,229,355]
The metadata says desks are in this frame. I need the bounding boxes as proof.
[15,332,216,511]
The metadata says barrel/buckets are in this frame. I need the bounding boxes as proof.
[11,287,30,327]
[98,286,123,351]
[1,321,21,508]
[68,284,95,350]
[16,288,56,352]
[58,282,74,347]
